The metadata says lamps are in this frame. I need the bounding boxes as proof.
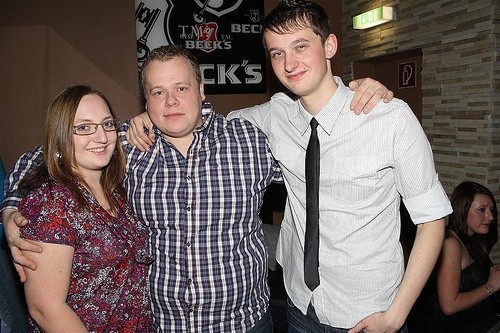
[353,7,396,30]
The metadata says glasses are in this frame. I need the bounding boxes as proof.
[72,118,121,135]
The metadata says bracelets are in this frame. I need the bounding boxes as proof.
[482,282,496,298]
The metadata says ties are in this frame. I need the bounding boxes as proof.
[304,117,321,292]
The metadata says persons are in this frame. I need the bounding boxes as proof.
[0,45,393,333]
[436,181,500,333]
[126,0,453,333]
[18,85,157,333]
